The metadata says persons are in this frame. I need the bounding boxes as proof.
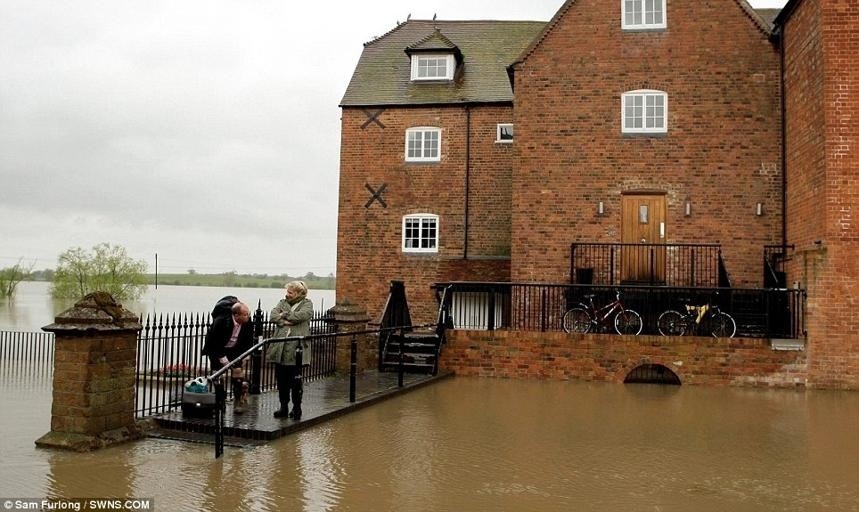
[204,302,254,415]
[266,281,314,419]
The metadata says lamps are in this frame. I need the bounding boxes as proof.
[684,202,691,216]
[756,201,763,216]
[598,201,604,215]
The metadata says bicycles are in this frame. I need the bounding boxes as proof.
[561,288,643,336]
[657,291,737,338]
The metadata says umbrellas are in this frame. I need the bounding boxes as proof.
[381,281,413,388]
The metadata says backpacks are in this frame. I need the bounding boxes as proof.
[211,296,239,319]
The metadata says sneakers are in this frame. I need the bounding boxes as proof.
[274,408,302,420]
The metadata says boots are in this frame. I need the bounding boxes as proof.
[232,377,246,415]
[211,378,227,412]
